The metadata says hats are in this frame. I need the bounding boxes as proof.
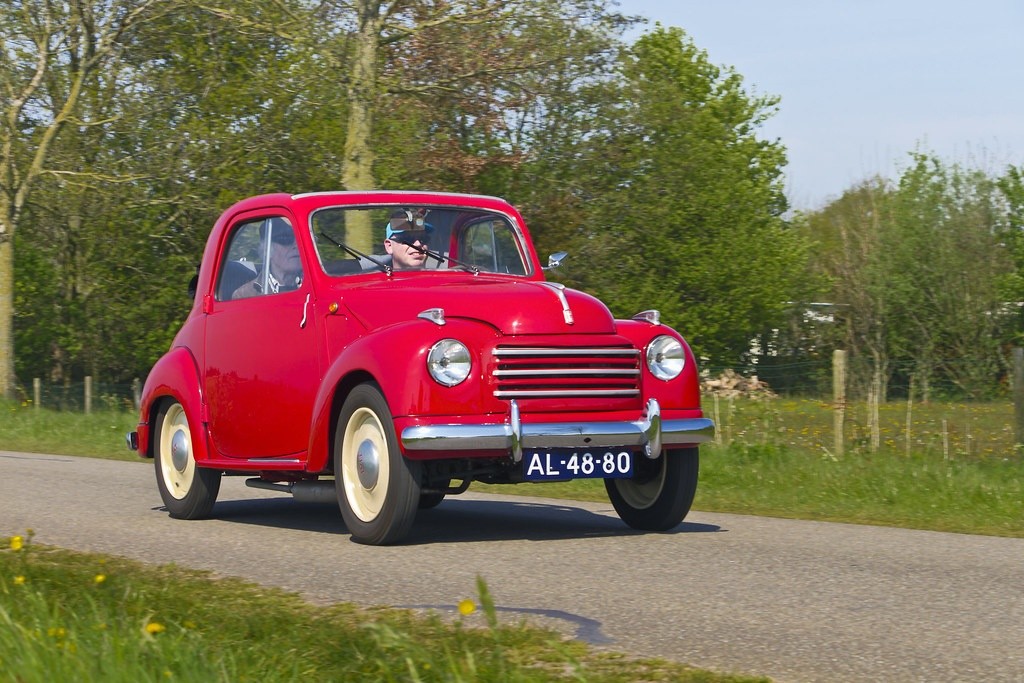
[385,217,435,239]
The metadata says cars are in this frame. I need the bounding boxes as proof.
[125,191,716,548]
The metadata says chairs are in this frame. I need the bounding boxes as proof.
[218,255,392,302]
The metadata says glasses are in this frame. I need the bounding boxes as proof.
[390,233,432,244]
[271,232,296,246]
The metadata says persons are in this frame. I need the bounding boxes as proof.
[232,217,303,299]
[384,218,436,272]
